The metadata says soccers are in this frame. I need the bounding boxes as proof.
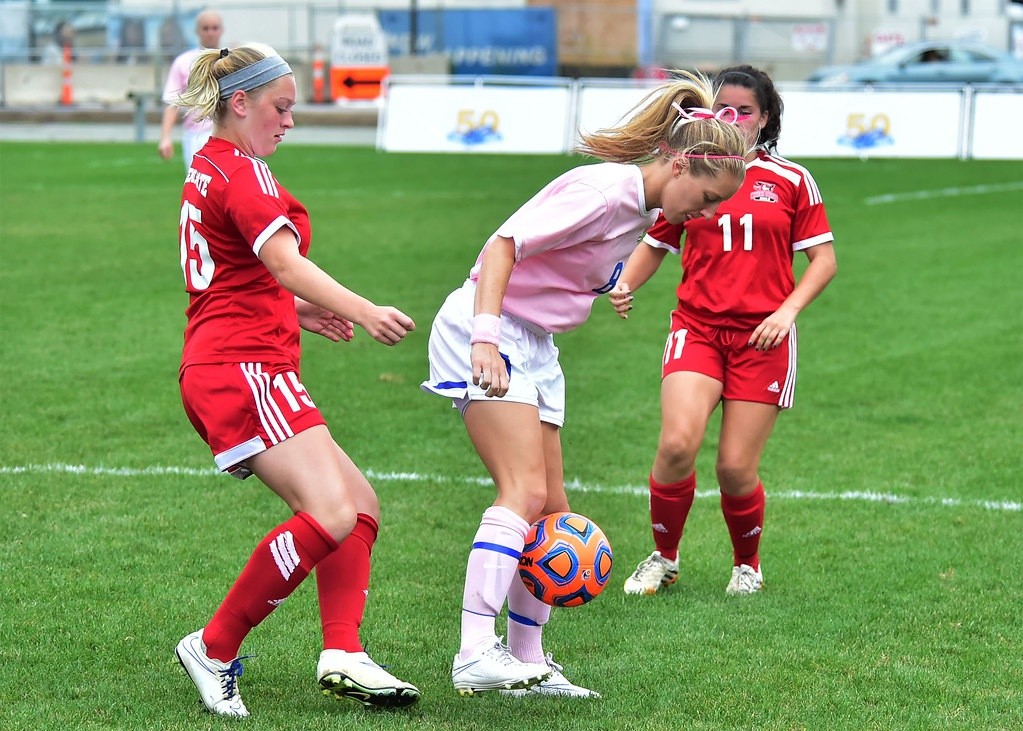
[519,512,612,608]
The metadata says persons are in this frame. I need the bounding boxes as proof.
[598,61,844,605]
[416,60,753,708]
[162,42,424,717]
[153,5,231,181]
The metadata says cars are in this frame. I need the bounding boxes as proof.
[808,28,1023,88]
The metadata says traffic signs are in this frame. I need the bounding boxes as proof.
[329,14,387,106]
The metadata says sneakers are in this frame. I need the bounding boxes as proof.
[724,563,763,596]
[173,628,251,719]
[623,549,680,595]
[452,643,552,696]
[499,662,603,700]
[314,649,422,708]
